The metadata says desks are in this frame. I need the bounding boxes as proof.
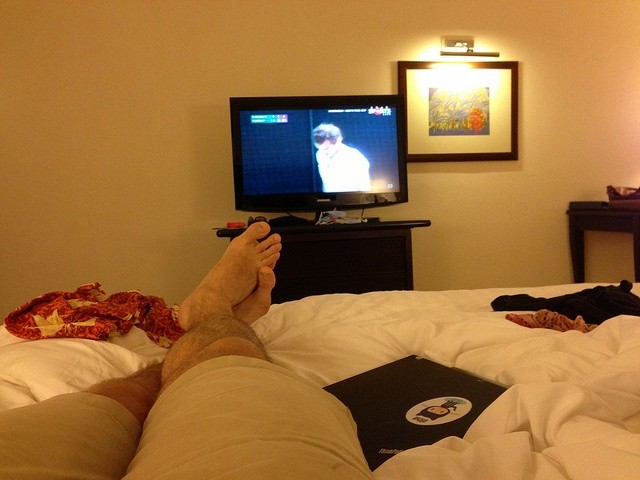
[566,201,639,284]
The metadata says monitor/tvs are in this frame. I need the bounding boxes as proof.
[228,94,409,224]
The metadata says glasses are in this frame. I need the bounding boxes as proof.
[313,135,340,145]
[247,216,267,228]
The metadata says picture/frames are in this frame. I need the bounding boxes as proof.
[396,59,519,163]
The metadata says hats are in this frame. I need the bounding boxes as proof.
[314,124,340,134]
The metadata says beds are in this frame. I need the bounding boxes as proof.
[1,280,640,479]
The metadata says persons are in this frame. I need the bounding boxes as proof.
[312,123,373,194]
[2,221,377,479]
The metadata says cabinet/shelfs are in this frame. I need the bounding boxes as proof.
[214,219,432,304]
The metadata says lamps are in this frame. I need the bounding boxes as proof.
[439,35,501,58]
